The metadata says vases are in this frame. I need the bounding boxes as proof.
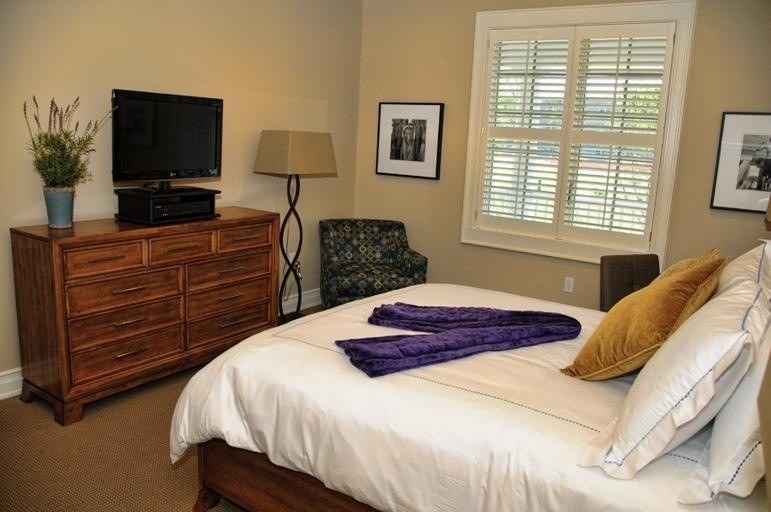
[42,186,74,229]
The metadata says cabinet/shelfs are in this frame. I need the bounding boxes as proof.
[9,205,279,427]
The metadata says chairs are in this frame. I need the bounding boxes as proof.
[601,253,661,311]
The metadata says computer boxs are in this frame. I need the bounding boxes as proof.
[113,185,221,226]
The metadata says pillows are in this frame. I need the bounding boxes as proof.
[560,257,728,380]
[576,237,769,480]
[643,246,720,286]
[674,329,769,507]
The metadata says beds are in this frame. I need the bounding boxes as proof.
[188,281,770,512]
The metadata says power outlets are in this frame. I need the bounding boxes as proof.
[563,277,574,293]
[284,252,298,275]
[563,276,575,294]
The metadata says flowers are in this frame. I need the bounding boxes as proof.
[22,95,116,186]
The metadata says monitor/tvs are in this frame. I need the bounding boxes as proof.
[111,88,224,194]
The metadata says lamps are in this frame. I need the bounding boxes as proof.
[253,131,338,324]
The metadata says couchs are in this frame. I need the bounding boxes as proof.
[318,217,427,304]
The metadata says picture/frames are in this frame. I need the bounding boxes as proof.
[706,111,768,216]
[375,102,444,181]
